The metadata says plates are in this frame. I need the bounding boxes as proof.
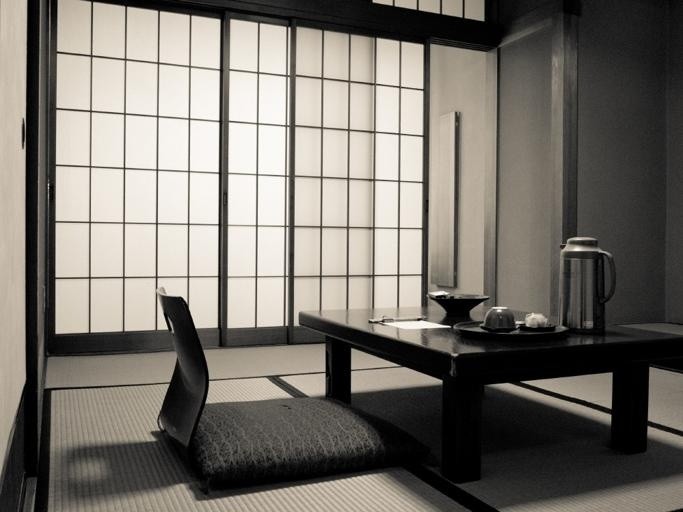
[452,321,570,339]
[480,323,518,332]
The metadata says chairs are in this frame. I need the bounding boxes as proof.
[152,285,390,491]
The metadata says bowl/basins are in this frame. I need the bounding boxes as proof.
[438,316,477,326]
[427,292,491,316]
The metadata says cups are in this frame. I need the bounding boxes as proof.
[483,306,516,329]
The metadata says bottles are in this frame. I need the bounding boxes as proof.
[559,234,616,331]
